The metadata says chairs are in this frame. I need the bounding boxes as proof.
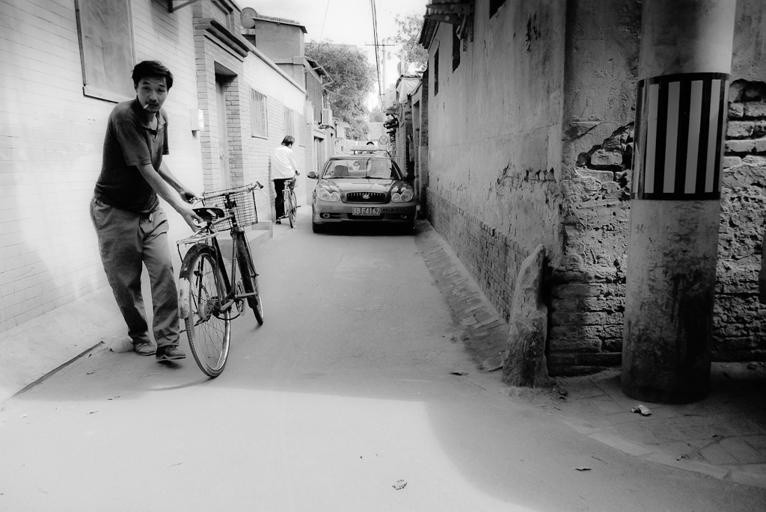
[333,165,349,176]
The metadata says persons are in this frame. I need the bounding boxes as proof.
[367,142,375,154]
[270,135,300,224]
[353,161,360,170]
[90,61,204,363]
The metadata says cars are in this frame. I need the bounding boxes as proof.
[308,154,418,238]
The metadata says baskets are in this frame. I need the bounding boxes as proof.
[202,183,258,231]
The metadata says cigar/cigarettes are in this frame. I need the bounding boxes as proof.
[144,104,150,109]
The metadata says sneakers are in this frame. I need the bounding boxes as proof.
[156,346,187,362]
[276,220,281,224]
[133,334,157,356]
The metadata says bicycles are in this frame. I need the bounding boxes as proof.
[174,180,266,379]
[276,170,302,232]
